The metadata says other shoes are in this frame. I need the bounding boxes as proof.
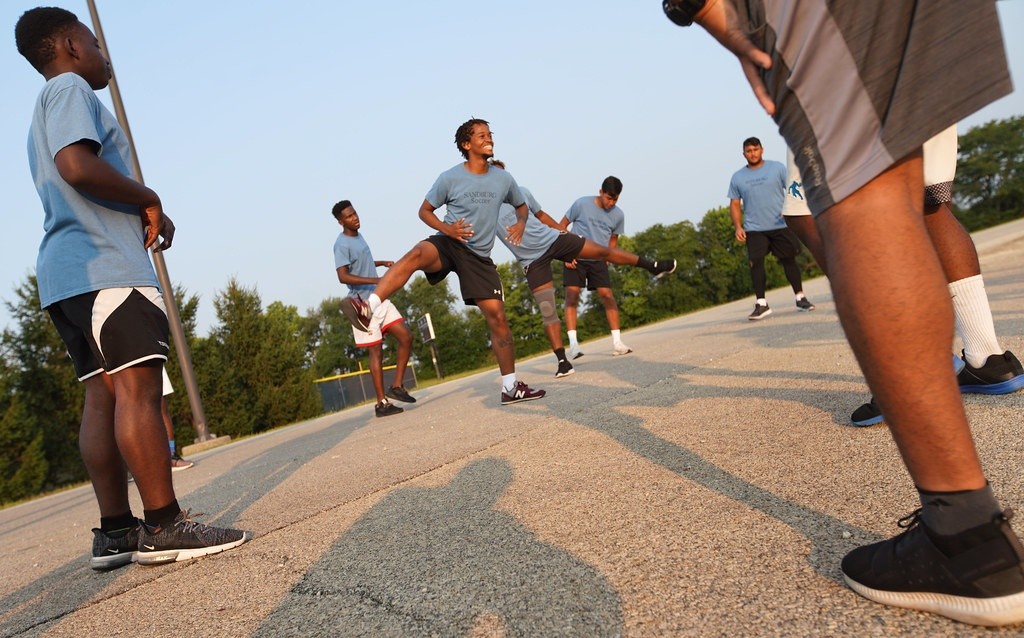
[127,472,134,482]
[171,453,194,472]
[613,345,632,356]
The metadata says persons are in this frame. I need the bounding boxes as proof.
[487,160,676,377]
[119,361,195,482]
[332,200,416,419]
[341,119,545,406]
[15,8,249,569]
[727,137,815,320]
[662,0,1024,625]
[557,176,632,359]
[782,117,1024,424]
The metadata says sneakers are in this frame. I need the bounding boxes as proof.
[749,303,771,320]
[375,399,403,417]
[340,296,373,331]
[650,259,677,279]
[571,349,585,359]
[840,508,1024,626]
[852,398,884,426]
[386,385,417,403]
[796,297,815,311]
[91,518,144,570]
[958,350,1024,394]
[501,380,545,405]
[556,360,574,378]
[137,506,247,565]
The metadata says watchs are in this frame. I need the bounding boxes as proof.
[662,0,707,26]
